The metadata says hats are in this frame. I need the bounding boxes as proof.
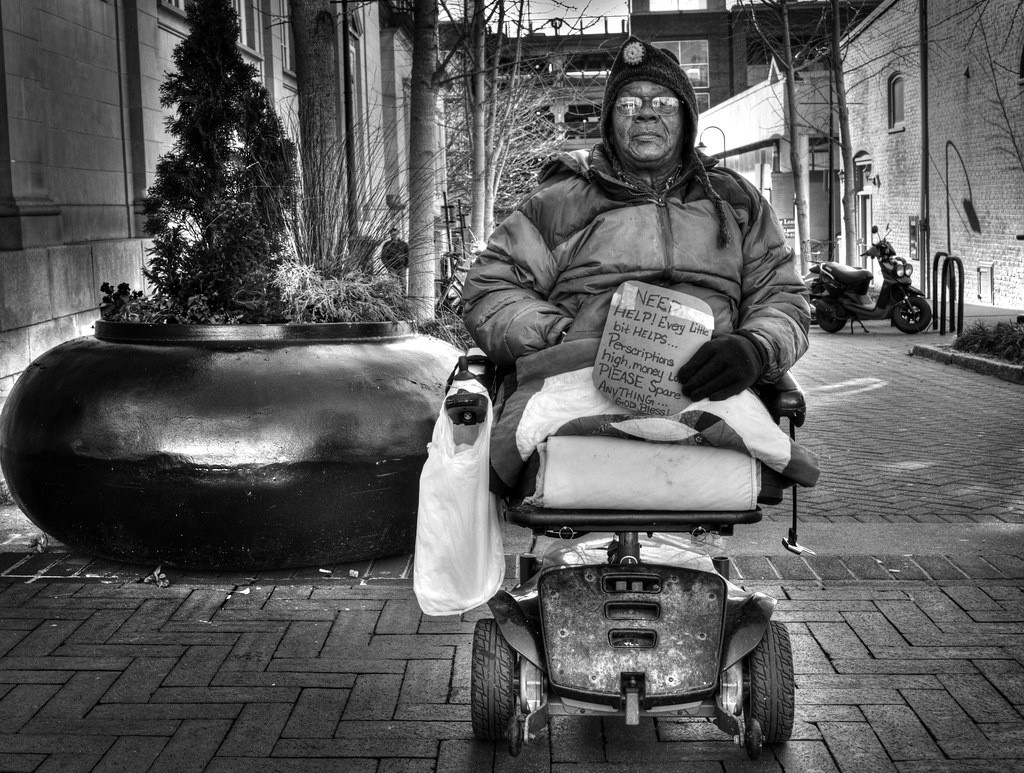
[600,36,730,250]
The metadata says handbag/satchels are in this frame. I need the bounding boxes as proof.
[413,383,506,616]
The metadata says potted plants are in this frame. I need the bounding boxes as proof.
[0,0,483,579]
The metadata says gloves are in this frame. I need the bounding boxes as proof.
[677,329,769,402]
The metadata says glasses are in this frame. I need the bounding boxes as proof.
[613,96,685,116]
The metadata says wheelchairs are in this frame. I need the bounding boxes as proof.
[443,347,807,760]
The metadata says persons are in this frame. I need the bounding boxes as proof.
[459,35,809,436]
[382,228,409,292]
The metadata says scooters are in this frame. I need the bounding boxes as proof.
[809,224,932,335]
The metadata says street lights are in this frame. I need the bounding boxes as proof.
[696,125,726,167]
